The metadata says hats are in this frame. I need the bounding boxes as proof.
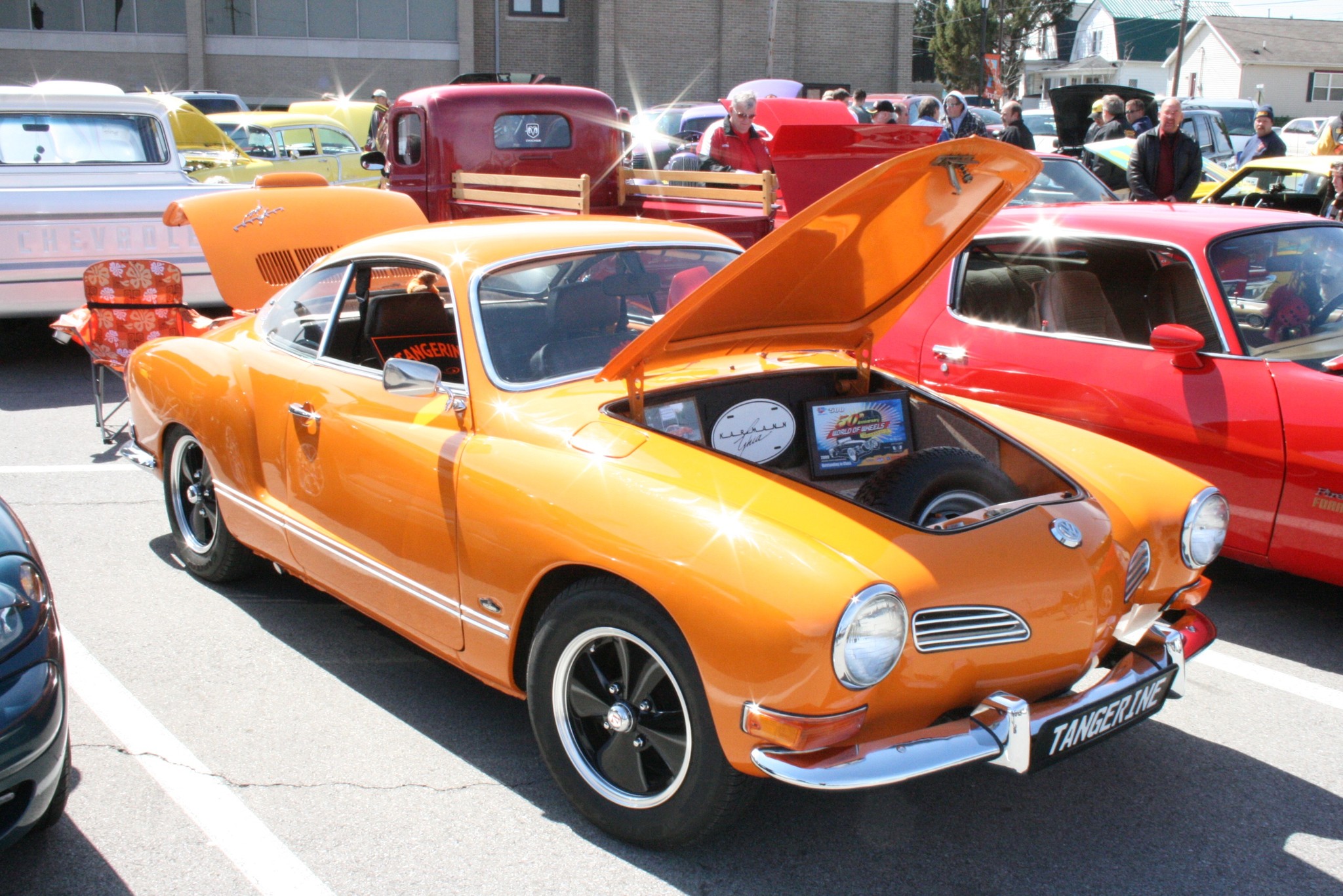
[1253,104,1274,123]
[1087,100,1104,118]
[822,90,833,99]
[865,100,896,114]
[371,89,387,98]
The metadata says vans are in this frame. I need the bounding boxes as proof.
[1153,96,1260,136]
[1048,84,1237,172]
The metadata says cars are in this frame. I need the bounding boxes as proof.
[621,78,1059,224]
[0,493,71,859]
[288,99,390,149]
[1083,136,1343,221]
[166,91,251,115]
[1279,116,1333,153]
[870,200,1343,588]
[125,139,1233,856]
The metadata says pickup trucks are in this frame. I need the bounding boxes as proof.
[0,78,263,323]
[359,71,778,324]
[129,92,387,188]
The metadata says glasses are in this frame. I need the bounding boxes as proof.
[944,102,961,108]
[1091,115,1102,121]
[1161,111,1181,117]
[1125,108,1139,114]
[732,107,756,120]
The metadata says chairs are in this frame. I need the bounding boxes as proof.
[1028,269,1125,340]
[48,260,216,445]
[530,283,637,377]
[363,292,455,377]
[1143,261,1223,350]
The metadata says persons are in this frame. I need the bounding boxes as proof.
[1235,104,1287,169]
[937,91,988,143]
[1320,163,1343,224]
[911,97,944,127]
[998,100,1036,151]
[1125,99,1153,139]
[1079,94,1203,202]
[1314,112,1343,156]
[365,88,392,152]
[697,91,779,190]
[821,88,909,124]
